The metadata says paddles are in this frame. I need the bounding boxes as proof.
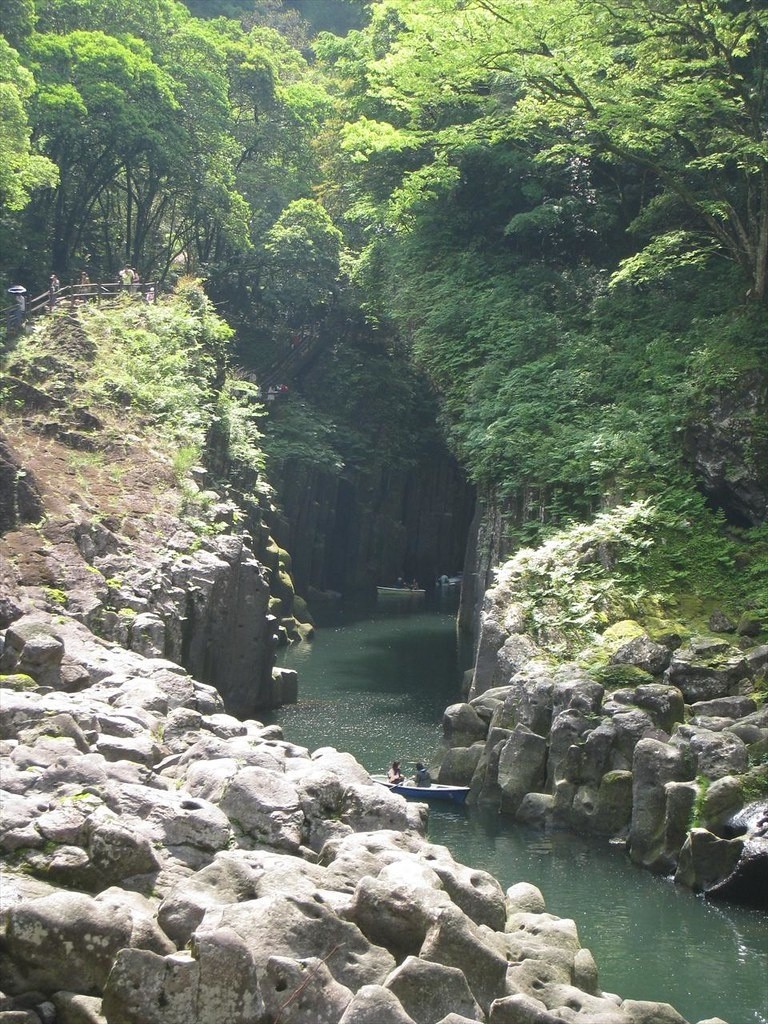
[388,775,415,790]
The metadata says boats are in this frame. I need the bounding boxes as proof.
[370,774,471,803]
[377,586,427,595]
[436,576,462,585]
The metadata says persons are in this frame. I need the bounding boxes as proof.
[438,575,449,583]
[121,265,134,295]
[50,274,60,305]
[147,288,155,300]
[15,292,25,313]
[414,762,431,788]
[411,578,420,589]
[130,268,139,293]
[79,271,90,303]
[387,761,405,785]
